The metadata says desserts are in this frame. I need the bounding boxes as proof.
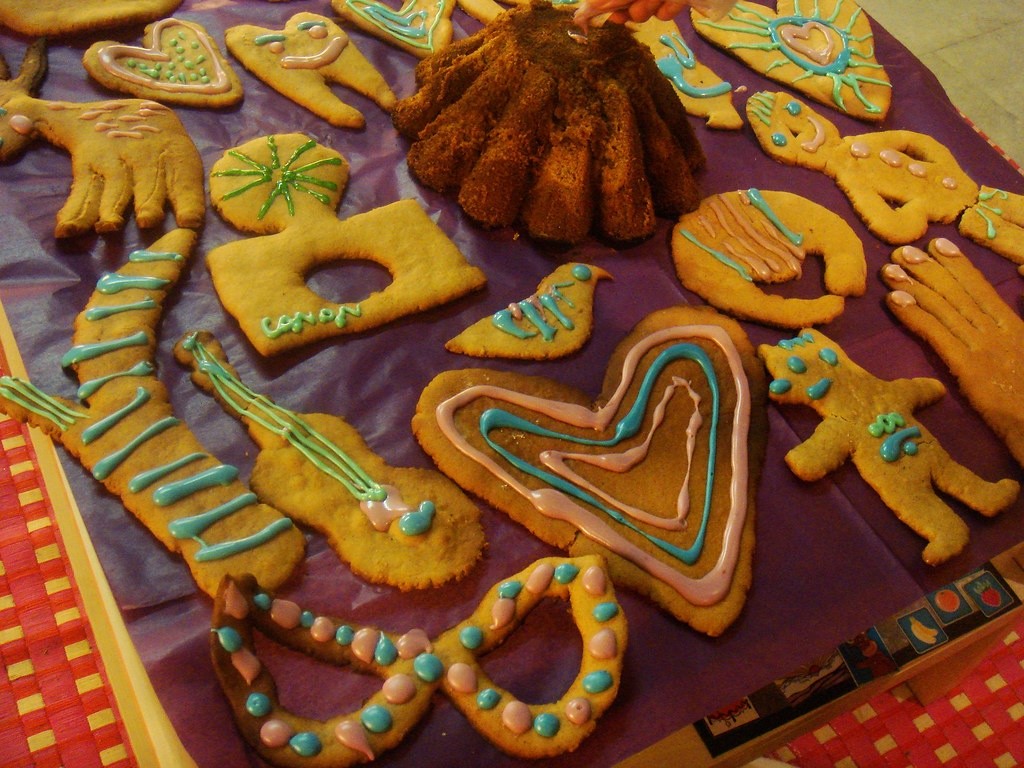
[0,1,1024,764]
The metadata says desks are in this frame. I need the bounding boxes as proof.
[0,0,1024,768]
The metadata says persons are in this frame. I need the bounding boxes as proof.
[573,0,690,26]
